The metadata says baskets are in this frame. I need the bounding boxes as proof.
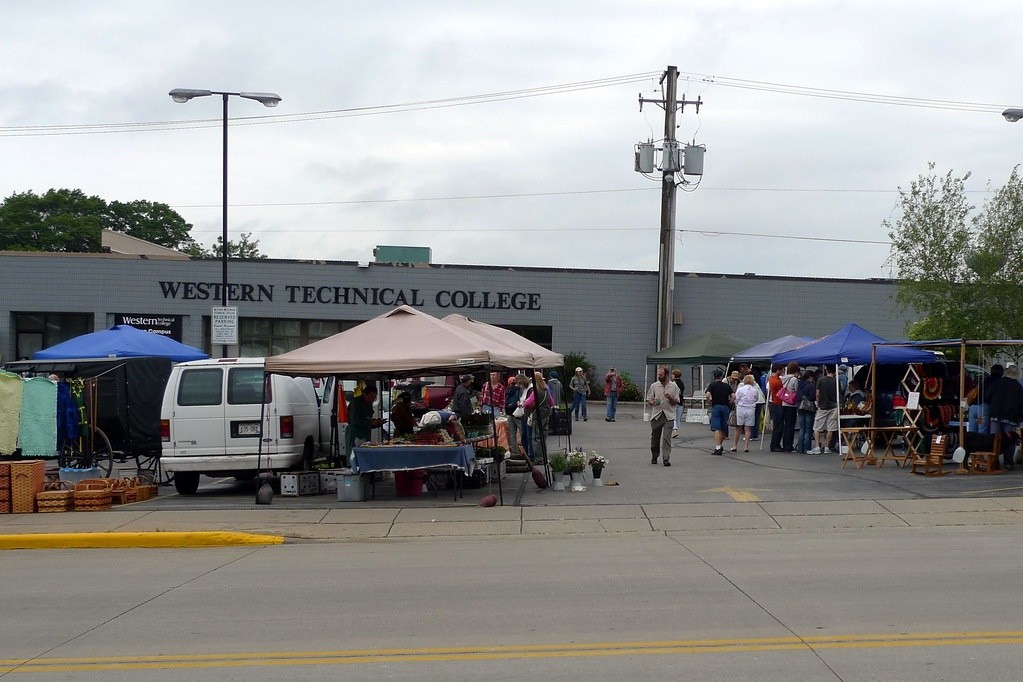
[0,459,158,513]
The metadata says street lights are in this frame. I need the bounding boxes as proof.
[168,88,283,359]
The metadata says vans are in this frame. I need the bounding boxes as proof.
[158,357,320,497]
[313,377,382,457]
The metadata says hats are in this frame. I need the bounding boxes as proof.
[731,371,739,379]
[575,367,583,372]
[838,364,847,369]
[549,372,558,379]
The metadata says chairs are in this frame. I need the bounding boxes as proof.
[909,434,947,476]
[970,434,1008,475]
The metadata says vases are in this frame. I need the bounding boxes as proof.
[592,468,602,479]
[572,473,582,482]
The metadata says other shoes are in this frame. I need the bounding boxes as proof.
[584,417,587,421]
[651,457,657,464]
[671,433,678,438]
[711,447,723,455]
[605,417,616,422]
[663,460,671,466]
[730,447,737,453]
[532,459,548,465]
[771,446,832,455]
[575,418,578,421]
[744,449,749,453]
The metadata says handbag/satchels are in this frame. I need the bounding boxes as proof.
[776,376,797,405]
[798,383,818,413]
[726,410,737,428]
[526,413,533,427]
[967,385,981,406]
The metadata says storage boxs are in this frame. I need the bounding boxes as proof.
[319,468,352,494]
[281,471,320,496]
[118,467,155,485]
[424,459,492,490]
[334,473,373,502]
[59,467,100,490]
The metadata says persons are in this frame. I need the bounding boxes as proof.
[968,363,1023,470]
[704,368,736,455]
[452,375,475,419]
[478,367,564,465]
[722,360,867,455]
[391,391,416,439]
[345,386,383,467]
[47,374,60,461]
[643,367,685,466]
[569,367,592,421]
[603,365,623,422]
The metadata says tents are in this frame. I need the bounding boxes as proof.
[32,324,209,451]
[868,336,1023,475]
[388,312,573,488]
[642,332,757,423]
[759,322,940,455]
[723,335,817,438]
[255,302,551,508]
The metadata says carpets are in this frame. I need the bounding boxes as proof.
[0,369,59,457]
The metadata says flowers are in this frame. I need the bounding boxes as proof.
[588,450,609,469]
[570,453,585,472]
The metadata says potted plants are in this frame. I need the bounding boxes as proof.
[563,461,571,482]
[477,446,507,481]
[548,402,572,435]
[548,452,566,491]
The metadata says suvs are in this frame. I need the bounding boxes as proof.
[852,360,992,399]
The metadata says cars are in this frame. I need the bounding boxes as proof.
[380,389,399,435]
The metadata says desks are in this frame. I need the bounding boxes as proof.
[949,421,967,426]
[839,414,871,439]
[840,426,920,472]
[453,432,499,448]
[349,444,477,502]
[683,396,711,409]
